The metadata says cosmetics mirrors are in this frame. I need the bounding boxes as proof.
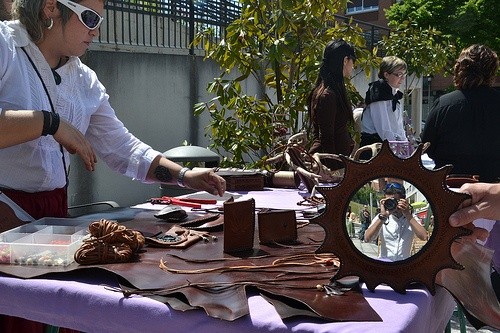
[311,139,474,296]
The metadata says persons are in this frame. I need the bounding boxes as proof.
[359,56,408,161]
[449,183,500,241]
[364,183,427,261]
[346,205,381,245]
[423,44,500,182]
[0,0,226,233]
[306,38,356,170]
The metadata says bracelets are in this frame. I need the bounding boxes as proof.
[177,167,190,187]
[379,214,387,220]
[47,112,60,135]
[41,110,50,136]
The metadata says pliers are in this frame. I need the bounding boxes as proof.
[146,196,218,208]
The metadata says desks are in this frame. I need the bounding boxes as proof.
[0,187,500,333]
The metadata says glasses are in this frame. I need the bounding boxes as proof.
[385,183,403,189]
[60,0,104,30]
[393,72,407,78]
[349,55,356,64]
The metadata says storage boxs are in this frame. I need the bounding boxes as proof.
[0,217,117,267]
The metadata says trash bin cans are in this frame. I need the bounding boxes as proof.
[159,145,220,200]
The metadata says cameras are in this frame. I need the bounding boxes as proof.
[382,198,398,211]
